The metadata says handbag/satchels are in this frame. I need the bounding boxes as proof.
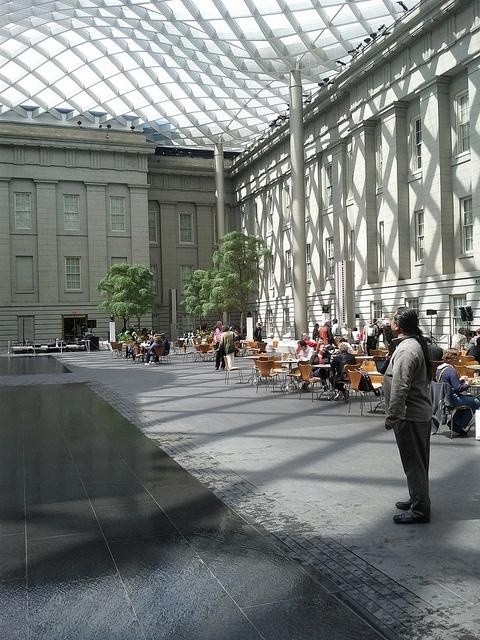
[358,371,373,391]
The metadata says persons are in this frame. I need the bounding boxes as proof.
[294,319,480,432]
[214,321,242,371]
[383,307,432,524]
[122,327,170,366]
[253,322,262,341]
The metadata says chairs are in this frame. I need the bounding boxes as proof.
[108,330,220,366]
[222,328,480,439]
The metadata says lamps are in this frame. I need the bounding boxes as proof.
[253,19,387,129]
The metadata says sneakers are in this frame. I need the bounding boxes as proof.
[393,512,429,523]
[396,500,411,510]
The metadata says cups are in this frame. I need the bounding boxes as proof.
[457,373,480,385]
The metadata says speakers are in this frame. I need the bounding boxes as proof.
[92,320,96,328]
[90,337,99,351]
[87,320,92,328]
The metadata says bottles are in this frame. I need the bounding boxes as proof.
[280,352,293,362]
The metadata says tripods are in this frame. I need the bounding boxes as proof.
[427,318,438,344]
